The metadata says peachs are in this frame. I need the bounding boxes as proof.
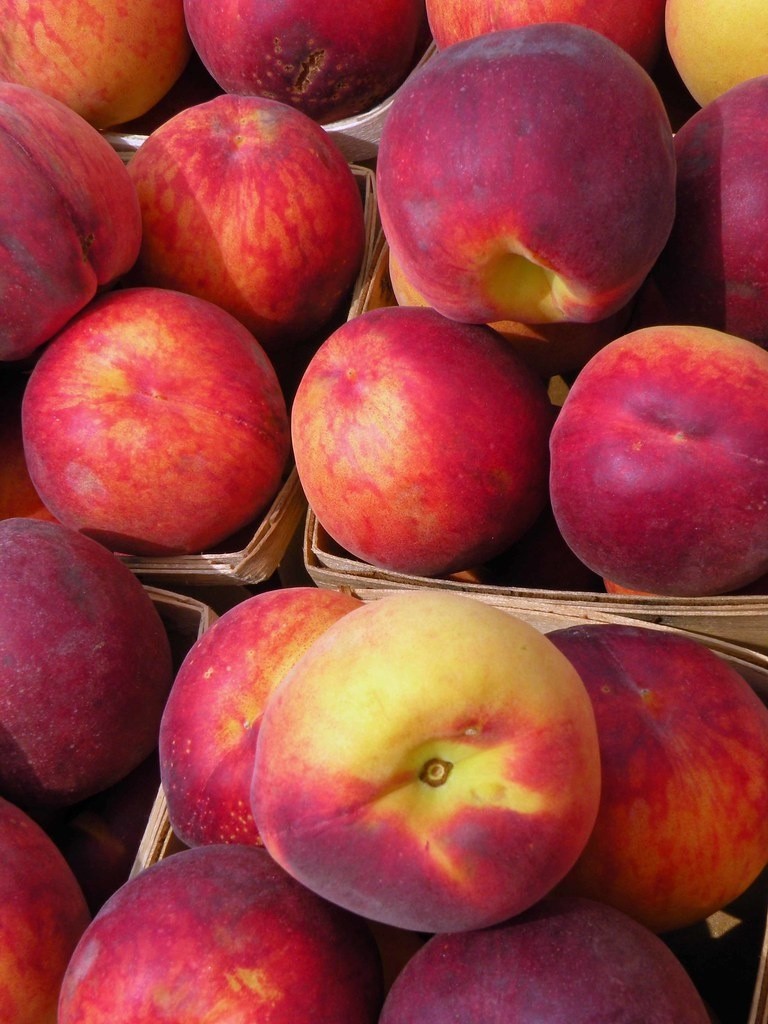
[0,0,768,1024]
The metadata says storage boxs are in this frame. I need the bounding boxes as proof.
[302,179,768,650]
[0,129,392,608]
[124,586,227,889]
